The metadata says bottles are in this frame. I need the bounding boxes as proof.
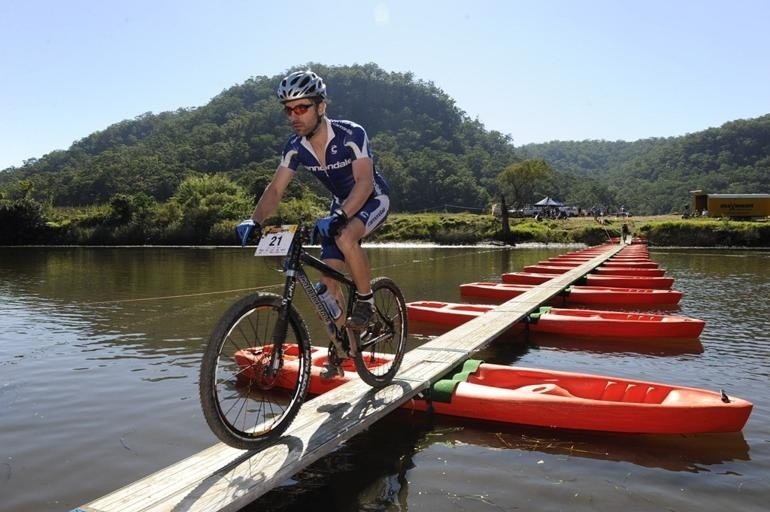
[313,280,343,324]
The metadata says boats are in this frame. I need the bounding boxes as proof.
[403,300,707,341]
[501,270,675,291]
[459,282,684,309]
[522,235,666,277]
[231,342,755,434]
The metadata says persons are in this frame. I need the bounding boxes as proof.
[621,224,629,244]
[693,208,701,218]
[233,68,393,385]
[534,202,611,223]
[619,203,627,218]
[681,207,690,220]
[701,207,710,216]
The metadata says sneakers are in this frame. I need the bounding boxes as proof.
[346,302,376,329]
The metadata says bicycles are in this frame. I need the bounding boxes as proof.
[200,223,408,450]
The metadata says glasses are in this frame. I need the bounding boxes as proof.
[284,104,316,115]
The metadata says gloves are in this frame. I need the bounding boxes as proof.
[310,214,348,246]
[235,220,264,248]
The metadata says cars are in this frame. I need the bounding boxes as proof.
[507,208,591,218]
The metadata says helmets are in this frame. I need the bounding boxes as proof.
[276,70,327,103]
